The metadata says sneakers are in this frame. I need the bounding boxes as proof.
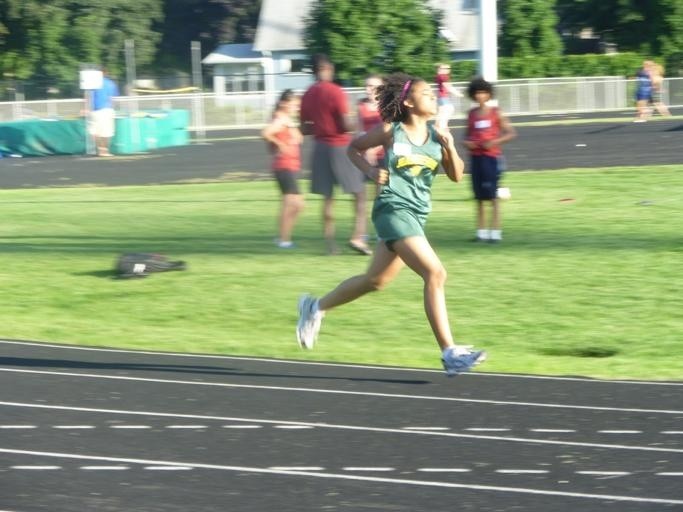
[347,241,372,254]
[471,237,500,244]
[297,294,321,351]
[273,241,301,250]
[440,346,486,377]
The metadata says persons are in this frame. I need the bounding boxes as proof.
[299,53,374,256]
[87,63,119,157]
[296,72,488,378]
[433,63,465,132]
[261,88,304,249]
[461,79,518,241]
[355,72,388,165]
[634,60,672,123]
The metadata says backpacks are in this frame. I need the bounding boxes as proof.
[116,252,188,278]
